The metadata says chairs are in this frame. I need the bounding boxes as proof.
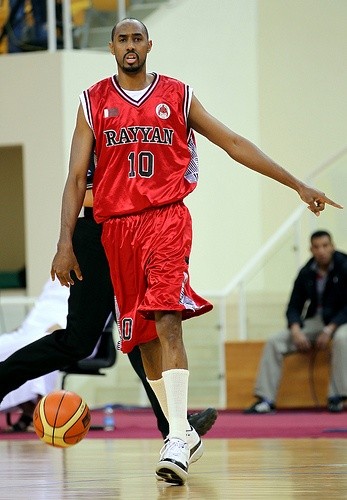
[6,310,116,432]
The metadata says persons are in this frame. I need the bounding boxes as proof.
[243,231,346,415]
[49,16,344,485]
[0,140,217,449]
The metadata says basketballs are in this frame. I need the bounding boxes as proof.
[33,391,91,449]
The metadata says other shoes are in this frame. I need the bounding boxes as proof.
[241,399,276,415]
[163,407,218,442]
[325,397,347,412]
[154,425,206,486]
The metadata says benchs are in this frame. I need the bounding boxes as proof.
[224,340,330,412]
